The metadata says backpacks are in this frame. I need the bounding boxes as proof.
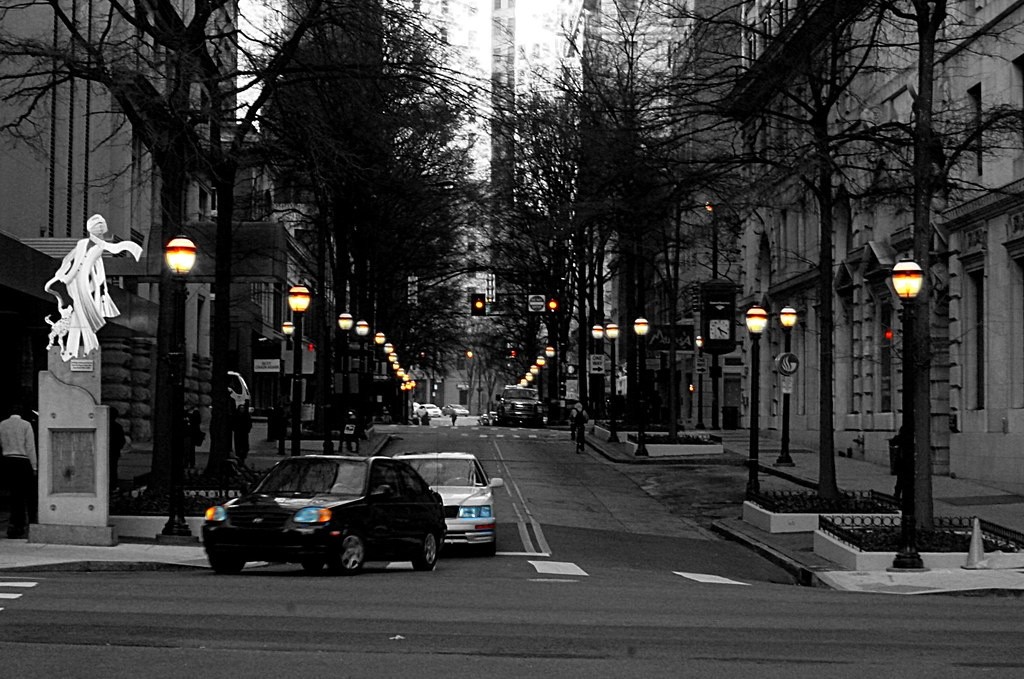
[574,407,585,428]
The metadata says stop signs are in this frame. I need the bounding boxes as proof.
[529,296,545,311]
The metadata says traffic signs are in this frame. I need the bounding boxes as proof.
[589,354,605,374]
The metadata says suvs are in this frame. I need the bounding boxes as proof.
[227,371,255,415]
[477,388,546,429]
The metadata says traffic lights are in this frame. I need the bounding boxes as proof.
[547,295,556,317]
[471,293,486,316]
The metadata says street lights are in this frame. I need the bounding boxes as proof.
[634,316,651,456]
[776,304,794,463]
[282,283,313,456]
[694,335,707,430]
[592,323,607,420]
[744,304,770,501]
[891,257,926,569]
[338,312,418,453]
[605,324,620,443]
[160,238,199,536]
[544,347,557,399]
[536,355,547,400]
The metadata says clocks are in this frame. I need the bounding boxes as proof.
[699,281,737,355]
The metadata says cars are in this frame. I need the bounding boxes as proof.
[415,404,442,418]
[201,453,449,578]
[393,451,506,559]
[545,398,581,426]
[442,404,470,417]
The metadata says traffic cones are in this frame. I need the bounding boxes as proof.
[959,518,994,570]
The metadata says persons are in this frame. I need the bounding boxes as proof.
[570,402,589,451]
[109,407,125,493]
[648,390,662,427]
[184,410,201,468]
[616,390,625,420]
[0,402,37,539]
[232,405,252,462]
[421,412,431,425]
